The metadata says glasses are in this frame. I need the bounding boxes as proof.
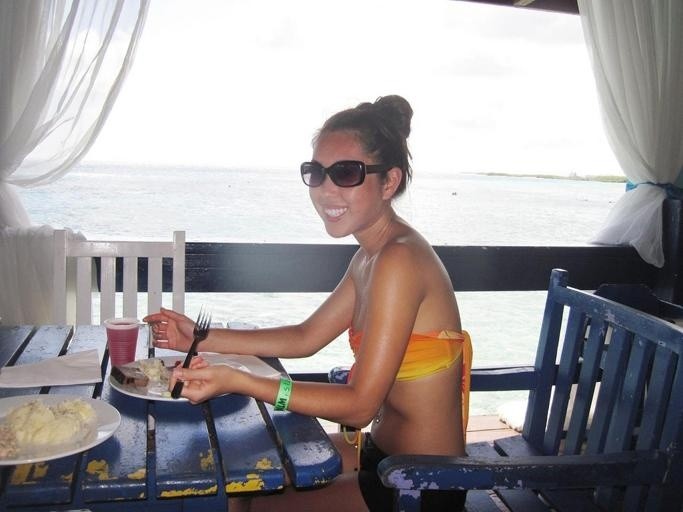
[300,160,389,188]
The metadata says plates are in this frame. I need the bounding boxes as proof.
[0,393,122,465]
[109,356,252,404]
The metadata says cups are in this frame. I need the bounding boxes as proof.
[104,318,141,368]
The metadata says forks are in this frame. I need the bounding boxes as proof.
[171,303,214,399]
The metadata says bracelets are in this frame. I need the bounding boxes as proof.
[274,377,293,412]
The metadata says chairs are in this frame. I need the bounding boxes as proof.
[53,231,185,326]
[329,267,683,511]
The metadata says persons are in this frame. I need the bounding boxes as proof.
[140,94,473,511]
